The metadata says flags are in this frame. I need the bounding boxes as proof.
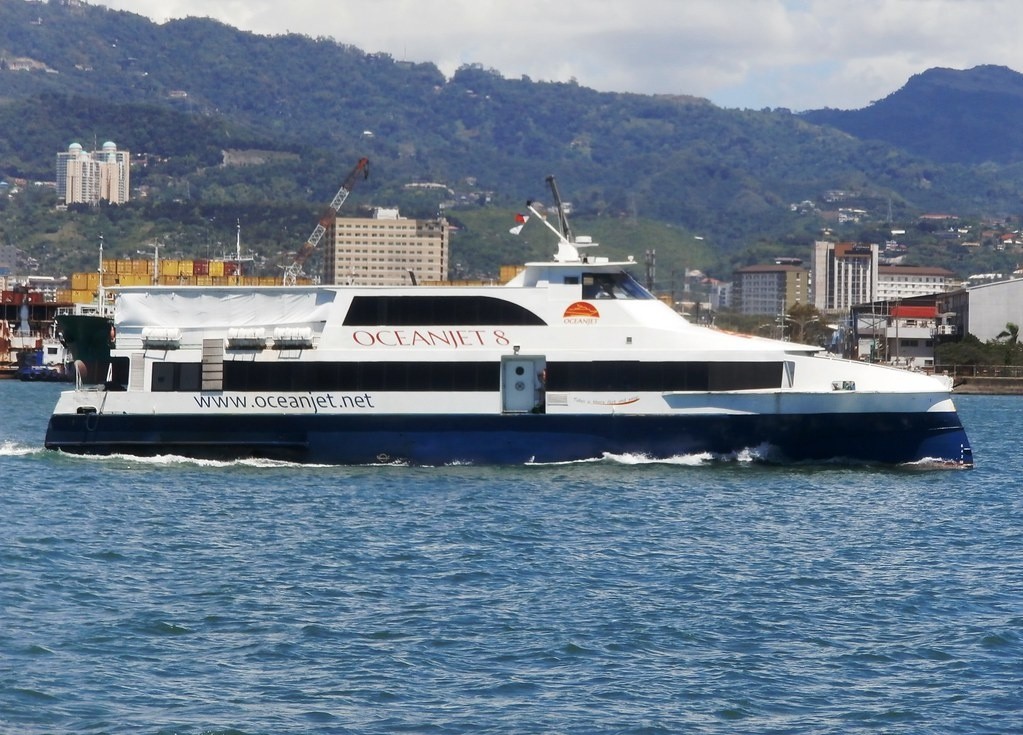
[508,213,530,236]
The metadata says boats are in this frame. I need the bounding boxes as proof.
[44,171,974,472]
[17,304,115,382]
[141,327,182,348]
[227,328,266,349]
[272,327,314,347]
